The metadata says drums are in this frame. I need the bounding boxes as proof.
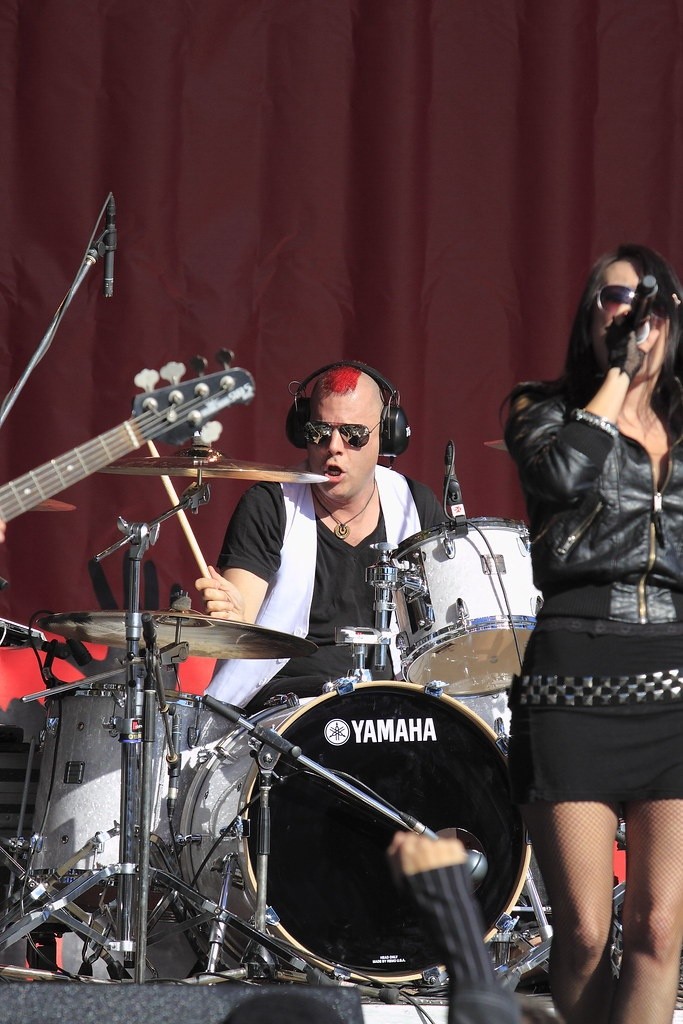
[179,681,533,990]
[21,681,263,912]
[378,514,546,696]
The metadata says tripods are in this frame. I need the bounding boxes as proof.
[0,466,332,993]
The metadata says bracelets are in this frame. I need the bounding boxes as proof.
[570,408,619,439]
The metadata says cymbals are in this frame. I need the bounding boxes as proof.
[94,457,328,484]
[35,611,319,658]
[29,499,77,511]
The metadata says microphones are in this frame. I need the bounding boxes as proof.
[400,813,488,891]
[443,443,463,507]
[66,637,92,665]
[167,712,183,816]
[105,195,116,298]
[626,273,659,344]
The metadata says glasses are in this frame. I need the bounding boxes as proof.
[302,420,381,449]
[596,283,679,320]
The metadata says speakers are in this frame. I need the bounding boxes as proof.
[0,980,364,1024]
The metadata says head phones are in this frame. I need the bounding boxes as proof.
[285,360,411,457]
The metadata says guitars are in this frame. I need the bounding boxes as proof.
[0,345,255,527]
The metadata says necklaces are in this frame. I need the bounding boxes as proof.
[311,479,375,539]
[499,244,683,1024]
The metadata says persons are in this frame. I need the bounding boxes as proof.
[194,357,446,720]
[221,832,520,1024]
